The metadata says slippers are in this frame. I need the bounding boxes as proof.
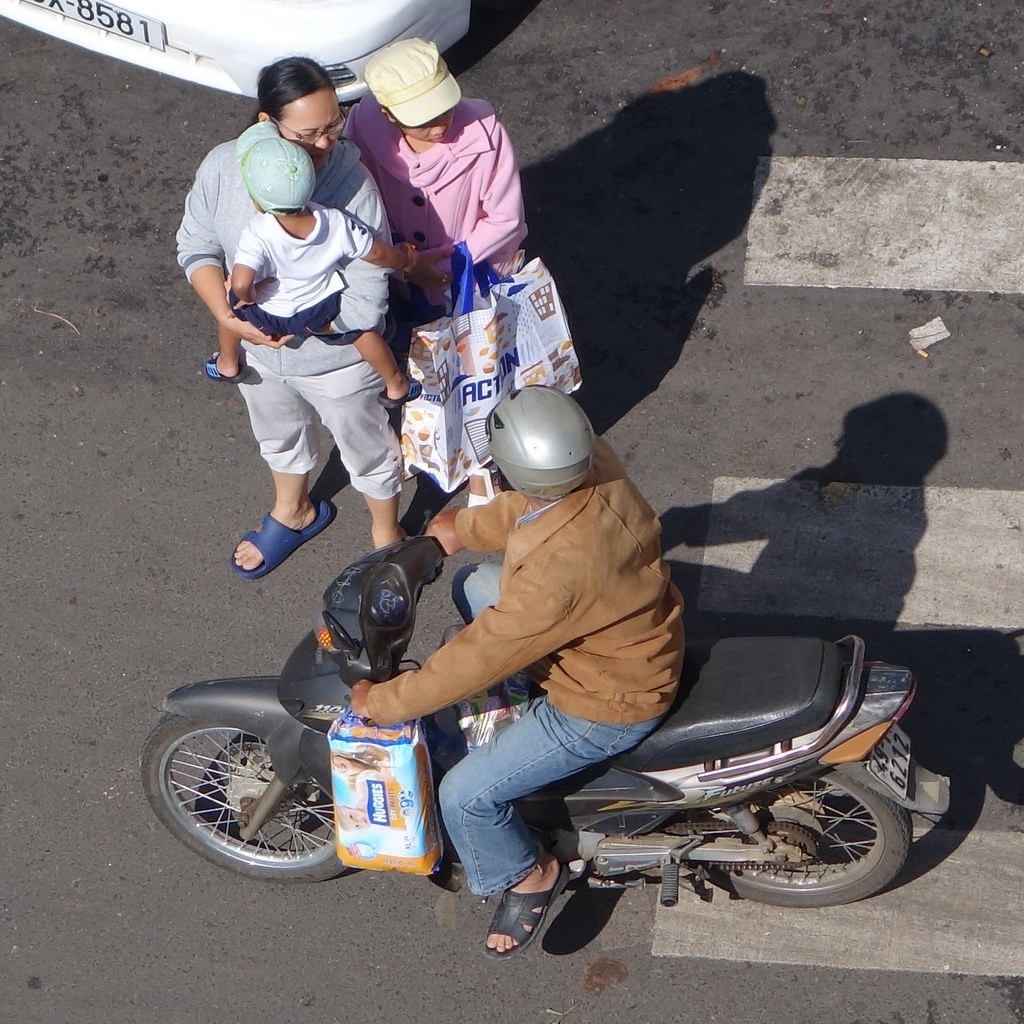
[230,495,335,580]
[480,854,572,964]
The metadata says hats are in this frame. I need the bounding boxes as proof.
[234,120,318,217]
[364,36,463,128]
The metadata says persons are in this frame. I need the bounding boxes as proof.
[351,384,686,960]
[175,37,529,580]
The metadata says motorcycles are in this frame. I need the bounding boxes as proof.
[137,532,952,906]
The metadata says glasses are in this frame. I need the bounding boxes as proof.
[269,107,348,148]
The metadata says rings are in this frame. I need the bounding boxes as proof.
[440,273,448,284]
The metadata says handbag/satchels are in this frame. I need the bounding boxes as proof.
[398,238,584,497]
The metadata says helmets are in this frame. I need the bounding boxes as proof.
[484,383,598,499]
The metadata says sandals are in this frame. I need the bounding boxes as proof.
[376,377,422,410]
[204,351,249,384]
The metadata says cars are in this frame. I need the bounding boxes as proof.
[0,0,472,105]
